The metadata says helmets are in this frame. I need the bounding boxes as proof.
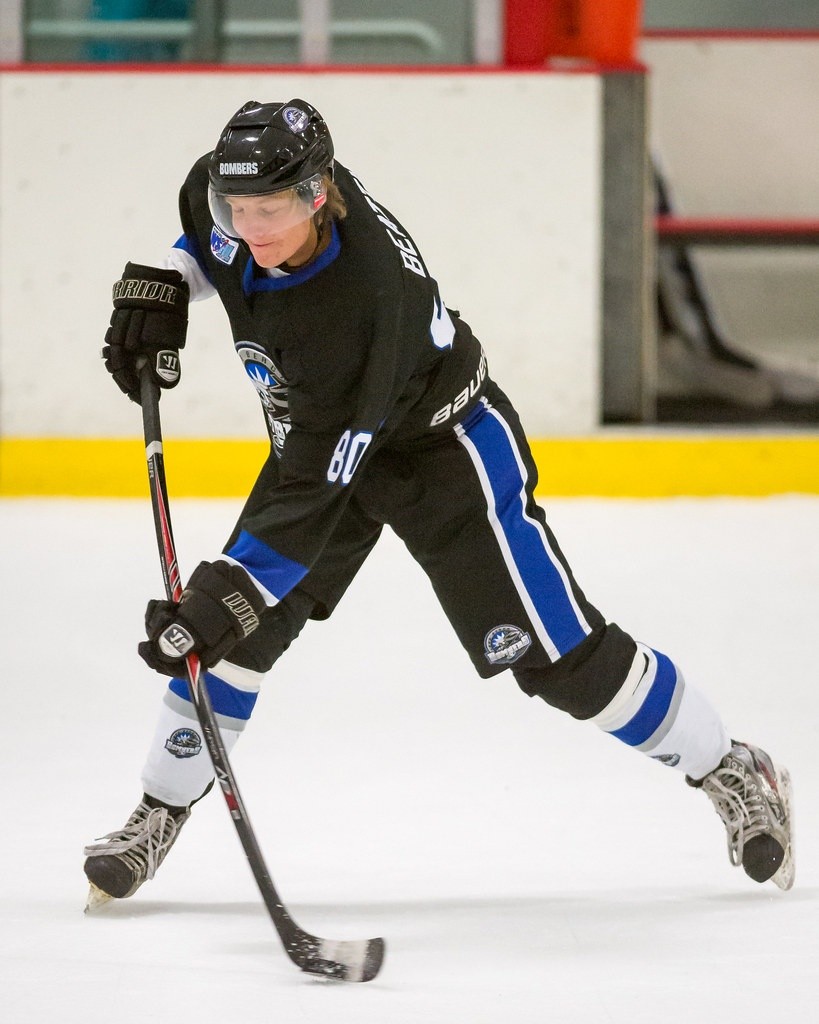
[207,98,334,195]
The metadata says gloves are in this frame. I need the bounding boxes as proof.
[138,559,269,678]
[102,262,191,405]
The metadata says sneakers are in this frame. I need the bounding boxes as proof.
[685,739,795,892]
[82,792,191,913]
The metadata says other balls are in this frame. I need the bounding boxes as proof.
[303,957,348,979]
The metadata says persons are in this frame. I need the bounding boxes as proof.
[85,98,795,912]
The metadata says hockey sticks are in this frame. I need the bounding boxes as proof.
[126,361,387,980]
